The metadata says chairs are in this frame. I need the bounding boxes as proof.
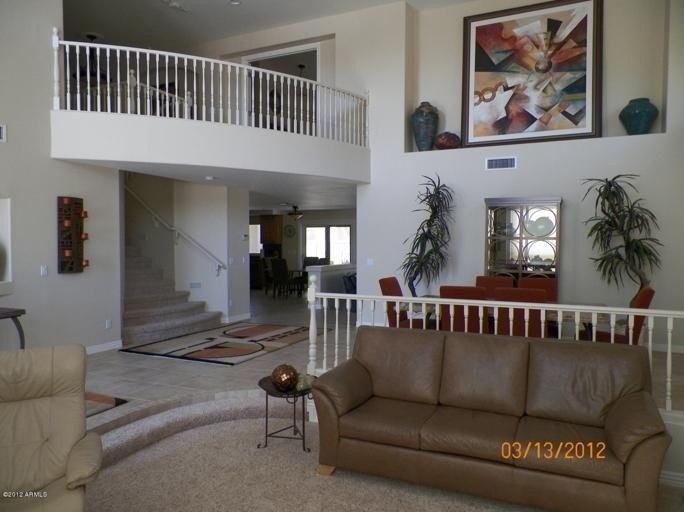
[378,276,655,345]
[264,270,308,301]
[0,343,102,512]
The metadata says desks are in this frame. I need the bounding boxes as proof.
[0,307,26,351]
[257,374,317,453]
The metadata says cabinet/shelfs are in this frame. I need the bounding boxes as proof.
[484,197,562,288]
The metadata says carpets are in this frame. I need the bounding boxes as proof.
[118,322,332,366]
[84,391,128,418]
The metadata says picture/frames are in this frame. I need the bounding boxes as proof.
[461,1,602,148]
[152,81,179,105]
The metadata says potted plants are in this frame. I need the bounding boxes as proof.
[580,173,663,346]
[395,171,457,319]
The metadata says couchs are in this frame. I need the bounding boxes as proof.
[311,325,672,512]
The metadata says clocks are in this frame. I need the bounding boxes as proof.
[284,224,296,237]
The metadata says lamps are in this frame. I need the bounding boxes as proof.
[57,196,90,274]
[288,206,303,216]
